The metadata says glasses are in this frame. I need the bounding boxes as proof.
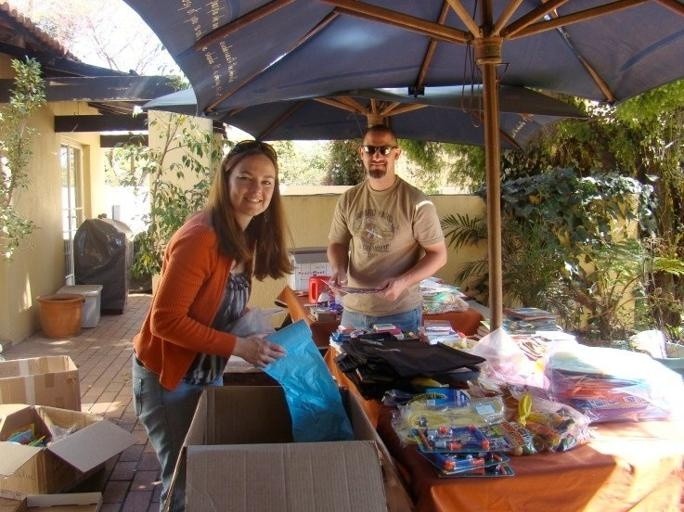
[226,139,278,162]
[361,144,398,156]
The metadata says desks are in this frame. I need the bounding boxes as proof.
[278,269,684,511]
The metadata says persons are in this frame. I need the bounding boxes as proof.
[323,123,446,338]
[127,142,297,512]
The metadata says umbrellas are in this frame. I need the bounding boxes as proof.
[123,0,683,332]
[134,84,592,147]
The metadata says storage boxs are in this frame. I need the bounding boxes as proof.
[2,402,141,500]
[164,384,394,512]
[15,491,106,512]
[55,282,103,329]
[1,352,82,413]
